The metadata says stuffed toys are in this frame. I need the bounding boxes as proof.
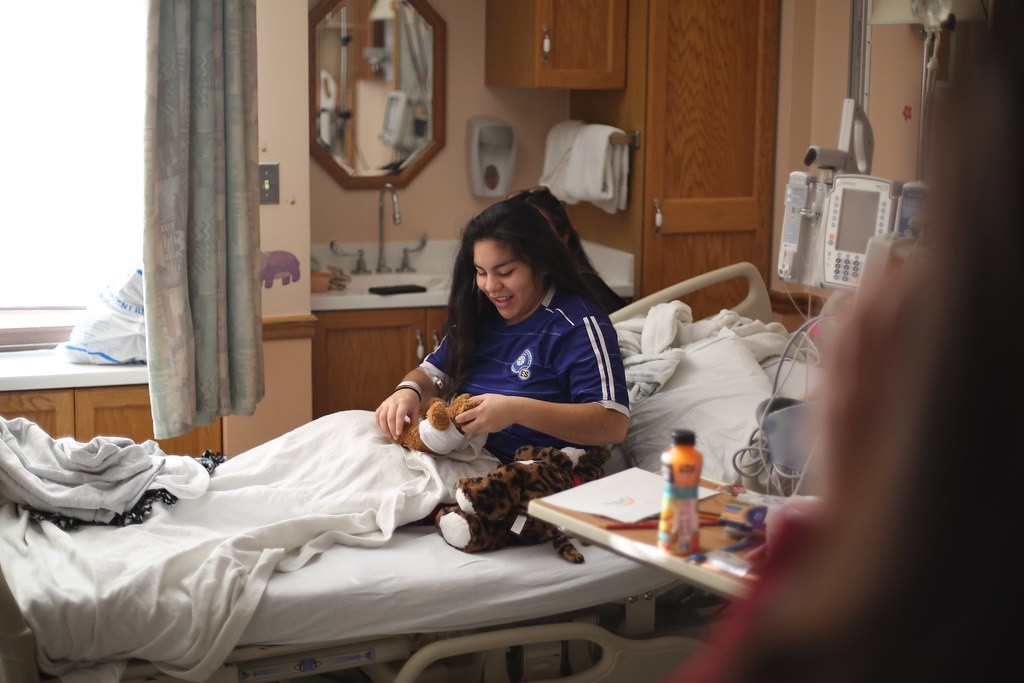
[433,444,611,563]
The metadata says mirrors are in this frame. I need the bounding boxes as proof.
[308,0,447,190]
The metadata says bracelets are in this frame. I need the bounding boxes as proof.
[393,381,422,402]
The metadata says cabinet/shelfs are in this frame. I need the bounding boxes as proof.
[0,386,221,459]
[567,1,782,323]
[484,0,627,90]
[312,307,448,419]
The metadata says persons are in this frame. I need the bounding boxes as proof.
[507,186,629,314]
[662,0,1024,683]
[374,199,630,526]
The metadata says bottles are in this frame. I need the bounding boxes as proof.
[658,431,704,557]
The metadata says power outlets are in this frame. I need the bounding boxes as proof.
[259,162,279,206]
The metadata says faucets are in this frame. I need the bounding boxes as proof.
[376,181,402,272]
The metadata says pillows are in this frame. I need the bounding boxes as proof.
[618,326,778,481]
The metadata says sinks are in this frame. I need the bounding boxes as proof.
[345,273,451,293]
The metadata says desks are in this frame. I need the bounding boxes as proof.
[527,470,765,597]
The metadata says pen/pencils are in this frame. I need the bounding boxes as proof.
[598,500,768,565]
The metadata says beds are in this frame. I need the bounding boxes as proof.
[0,263,817,683]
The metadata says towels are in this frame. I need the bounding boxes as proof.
[0,415,210,521]
[538,119,630,216]
[604,299,806,404]
